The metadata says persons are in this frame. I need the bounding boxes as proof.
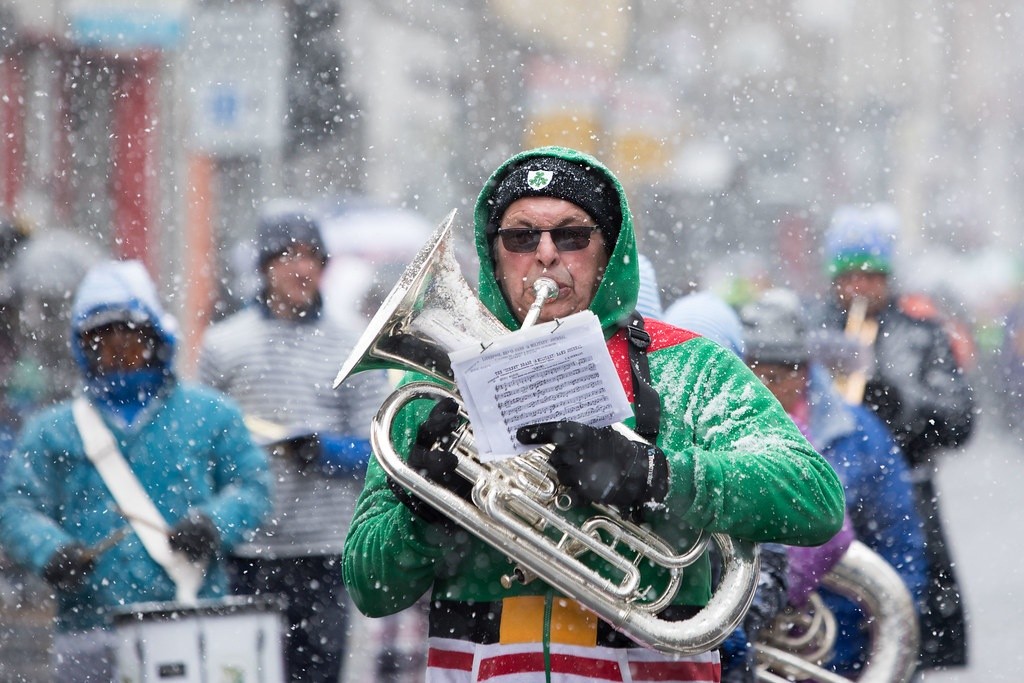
[201,210,391,683]
[0,260,272,683]
[362,204,977,683]
[343,147,847,683]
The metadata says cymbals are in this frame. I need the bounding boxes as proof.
[243,413,316,448]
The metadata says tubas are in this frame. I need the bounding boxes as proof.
[748,533,920,682]
[329,204,761,661]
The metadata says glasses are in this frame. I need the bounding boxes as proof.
[496,224,600,253]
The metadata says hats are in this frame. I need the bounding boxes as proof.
[257,212,329,271]
[830,252,891,281]
[486,156,620,263]
[662,292,745,360]
[636,254,661,320]
[78,309,150,331]
[740,288,814,363]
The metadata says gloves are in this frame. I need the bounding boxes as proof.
[168,514,219,563]
[42,543,93,589]
[515,420,669,527]
[385,397,462,524]
[286,433,319,471]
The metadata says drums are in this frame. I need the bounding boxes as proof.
[109,593,289,683]
